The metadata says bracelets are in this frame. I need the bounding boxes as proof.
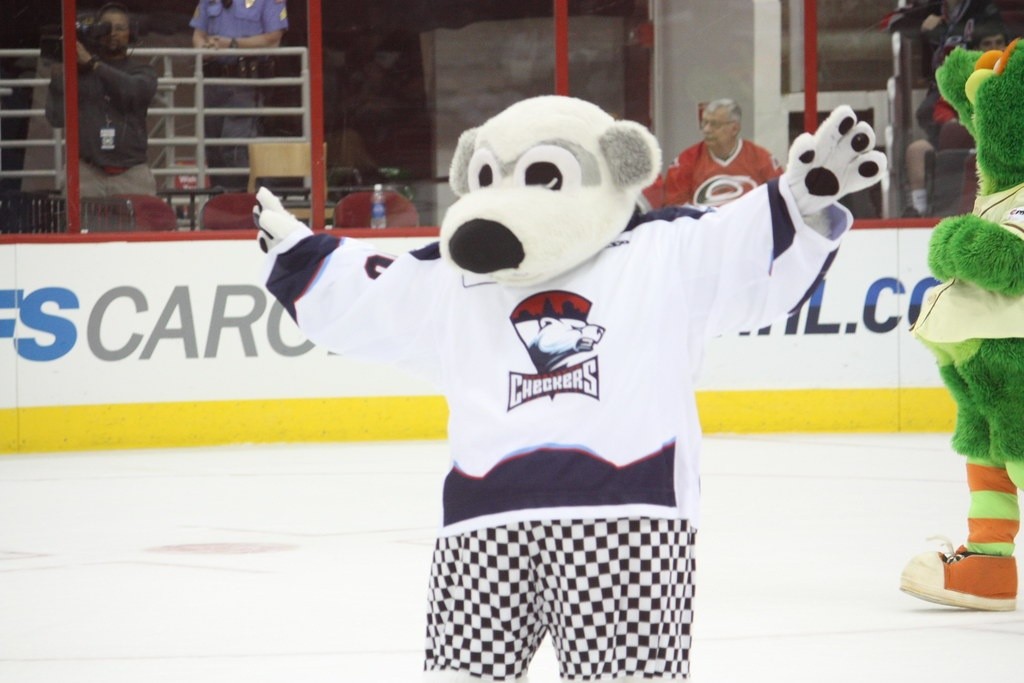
[229,37,237,48]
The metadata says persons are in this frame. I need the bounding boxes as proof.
[45,4,157,196]
[890,0,1024,217]
[630,97,784,211]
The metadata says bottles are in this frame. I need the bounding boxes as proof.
[370,183,386,229]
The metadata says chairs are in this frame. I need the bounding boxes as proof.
[248,142,335,222]
[90,195,176,233]
[200,194,257,230]
[927,120,979,217]
[334,190,419,227]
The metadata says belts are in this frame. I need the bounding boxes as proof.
[85,158,128,174]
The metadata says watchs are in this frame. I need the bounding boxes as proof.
[188,0,290,191]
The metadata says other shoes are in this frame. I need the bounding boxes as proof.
[902,204,934,217]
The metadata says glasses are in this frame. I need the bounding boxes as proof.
[700,118,729,129]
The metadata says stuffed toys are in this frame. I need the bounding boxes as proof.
[252,94,887,683]
[898,38,1024,613]
